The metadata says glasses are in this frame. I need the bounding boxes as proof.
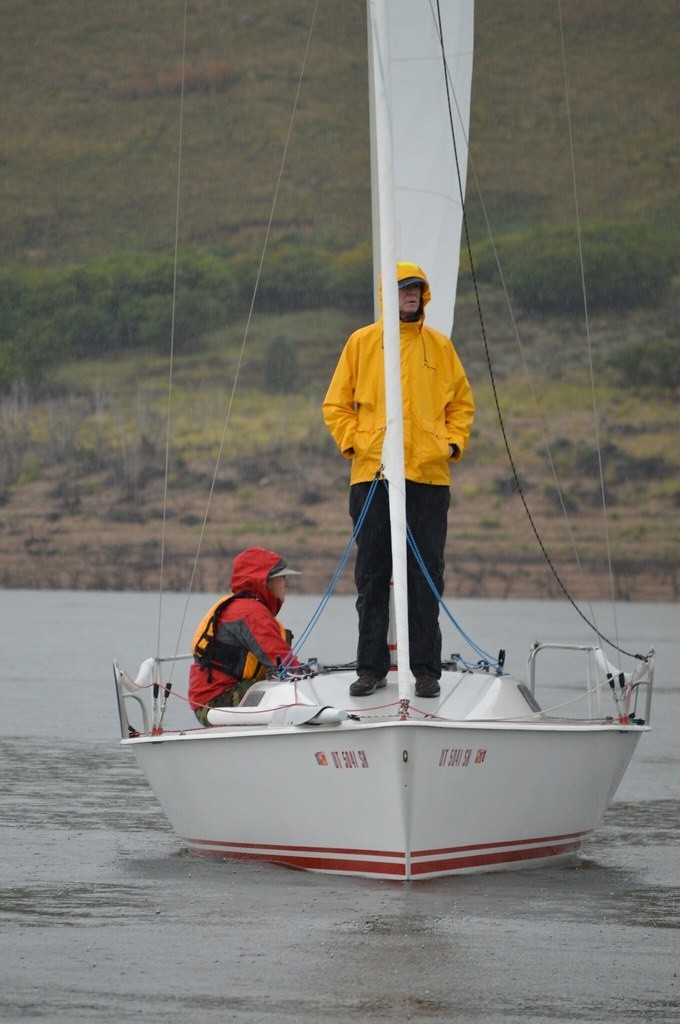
[267,575,287,582]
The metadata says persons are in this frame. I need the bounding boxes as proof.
[322,262,475,696]
[189,547,317,726]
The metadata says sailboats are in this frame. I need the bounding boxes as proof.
[112,0,654,884]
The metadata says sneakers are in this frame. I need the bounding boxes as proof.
[350,674,387,696]
[415,676,441,697]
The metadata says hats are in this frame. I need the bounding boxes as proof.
[398,277,426,289]
[267,559,301,579]
